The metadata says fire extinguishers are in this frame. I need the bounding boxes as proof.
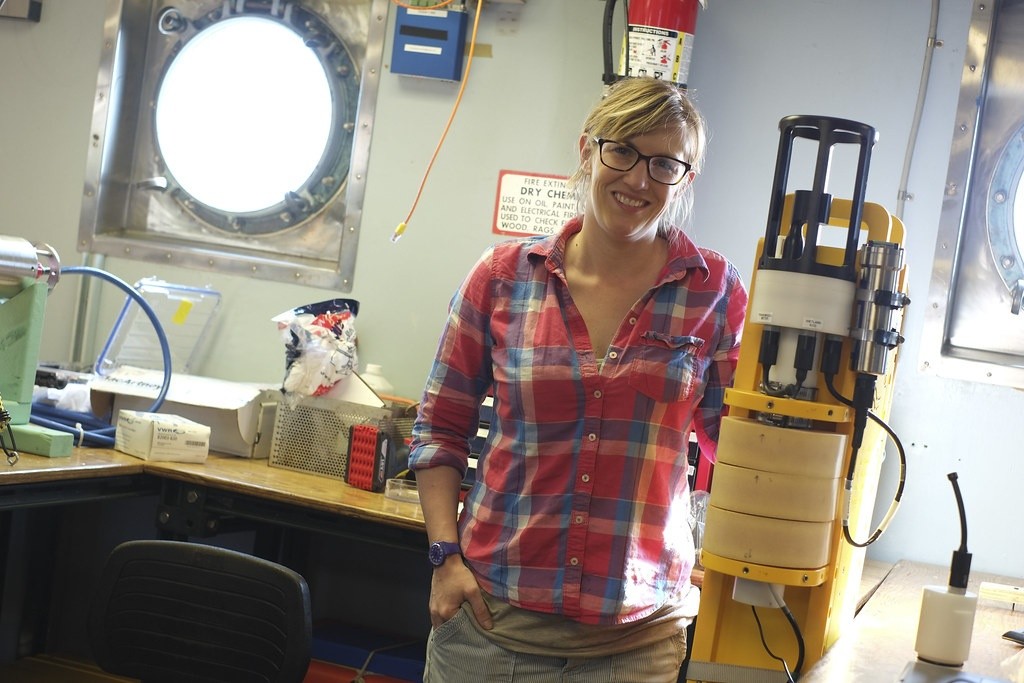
[601,0,707,98]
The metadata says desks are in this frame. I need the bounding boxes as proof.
[0,441,461,642]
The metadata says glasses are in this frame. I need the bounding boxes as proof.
[593,136,692,185]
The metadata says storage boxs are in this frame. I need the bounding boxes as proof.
[114,410,211,463]
[89,367,283,459]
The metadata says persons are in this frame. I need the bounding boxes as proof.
[407,75,749,683]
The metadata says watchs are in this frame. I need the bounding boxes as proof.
[428,541,460,567]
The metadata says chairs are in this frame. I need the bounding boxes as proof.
[90,539,315,683]
